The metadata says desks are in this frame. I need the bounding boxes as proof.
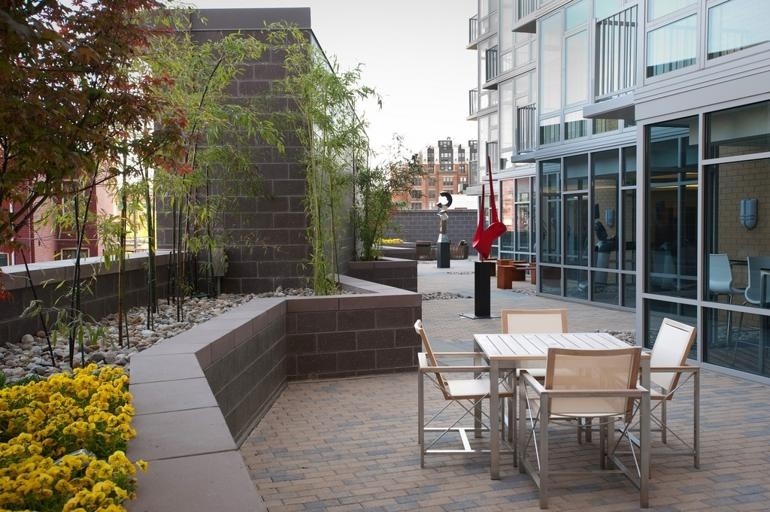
[729,259,748,268]
[757,267,770,375]
[473,331,653,482]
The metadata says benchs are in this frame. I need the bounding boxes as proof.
[496,257,536,291]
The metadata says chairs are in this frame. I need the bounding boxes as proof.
[584,313,703,478]
[499,309,583,444]
[413,317,518,481]
[705,253,760,349]
[731,256,770,367]
[517,342,655,511]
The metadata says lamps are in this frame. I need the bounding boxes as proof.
[739,197,758,231]
[604,208,615,227]
